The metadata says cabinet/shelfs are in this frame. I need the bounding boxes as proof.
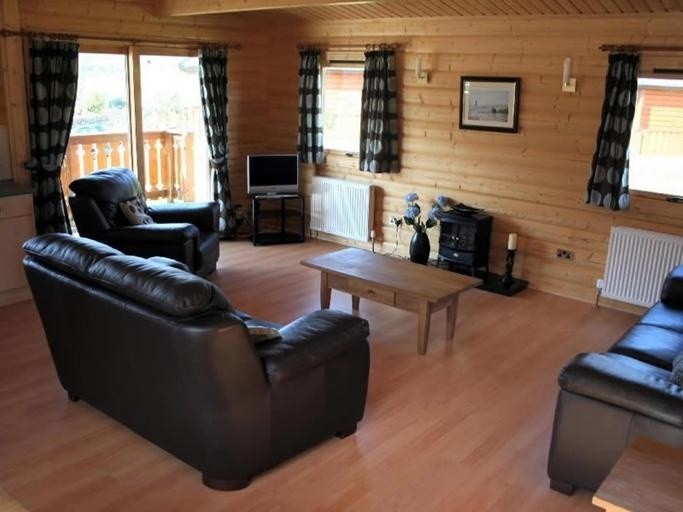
[436,209,492,282]
[253,194,304,245]
[1,181,38,307]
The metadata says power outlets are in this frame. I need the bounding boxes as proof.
[391,216,401,228]
[557,248,575,262]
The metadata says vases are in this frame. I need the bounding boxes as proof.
[410,233,430,265]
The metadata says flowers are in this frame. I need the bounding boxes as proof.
[403,192,448,234]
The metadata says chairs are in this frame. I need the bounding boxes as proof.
[68,166,219,280]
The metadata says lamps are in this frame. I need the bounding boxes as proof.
[561,58,576,92]
[415,60,429,84]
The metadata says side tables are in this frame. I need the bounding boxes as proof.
[592,434,683,512]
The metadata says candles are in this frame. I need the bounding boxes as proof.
[507,232,518,250]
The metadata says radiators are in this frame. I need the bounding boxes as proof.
[591,226,683,309]
[310,175,375,243]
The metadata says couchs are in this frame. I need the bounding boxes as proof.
[22,233,369,490]
[548,261,682,496]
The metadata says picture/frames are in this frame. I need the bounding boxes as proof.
[458,76,520,132]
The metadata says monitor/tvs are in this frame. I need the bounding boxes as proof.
[247,154,299,199]
